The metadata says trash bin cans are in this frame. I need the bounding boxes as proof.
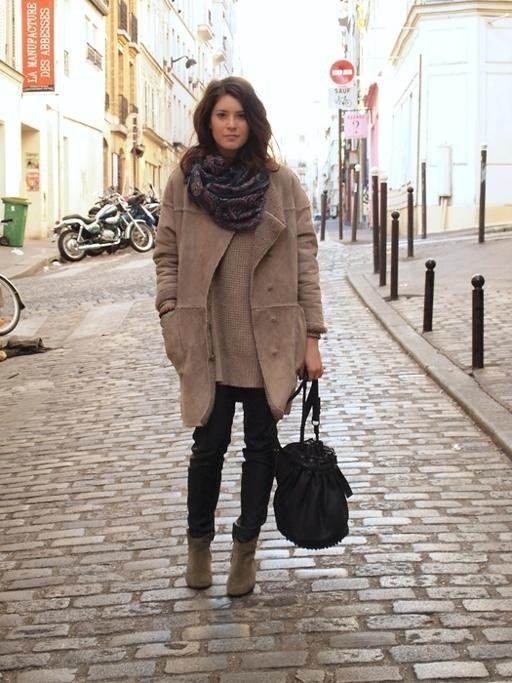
[0,197,31,247]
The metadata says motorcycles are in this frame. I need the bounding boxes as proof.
[53,181,162,262]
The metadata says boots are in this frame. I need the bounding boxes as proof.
[227,537,257,596]
[185,529,214,589]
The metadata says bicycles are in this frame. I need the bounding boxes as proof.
[0,217,25,337]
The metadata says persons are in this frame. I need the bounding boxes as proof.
[151,75,327,598]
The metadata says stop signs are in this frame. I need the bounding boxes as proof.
[330,58,356,84]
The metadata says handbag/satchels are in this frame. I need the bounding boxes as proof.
[273,438,352,550]
